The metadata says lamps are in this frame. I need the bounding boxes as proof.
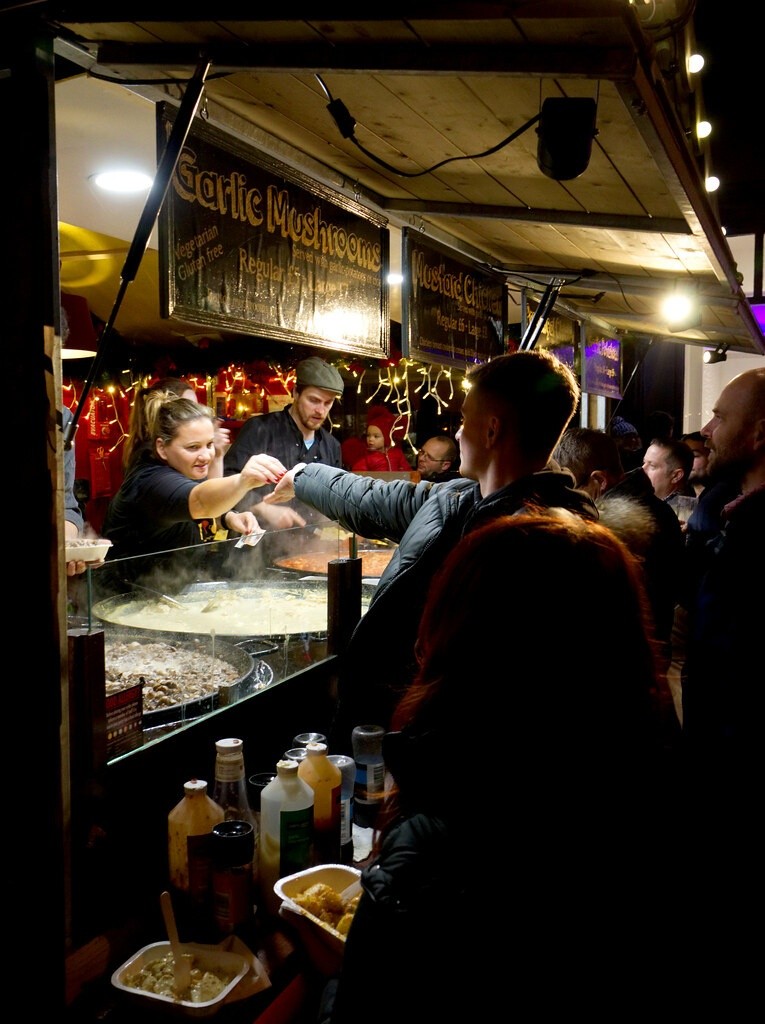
[536,98,600,180]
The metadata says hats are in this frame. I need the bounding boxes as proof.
[613,416,639,439]
[297,357,345,395]
[366,406,396,449]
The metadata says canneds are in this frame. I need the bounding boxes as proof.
[209,818,255,932]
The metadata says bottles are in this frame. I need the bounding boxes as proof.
[210,820,256,937]
[212,738,277,900]
[259,758,316,911]
[296,742,341,865]
[284,725,387,865]
[168,779,225,918]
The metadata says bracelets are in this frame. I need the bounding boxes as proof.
[221,510,239,530]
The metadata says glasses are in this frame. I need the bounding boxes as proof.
[417,447,450,464]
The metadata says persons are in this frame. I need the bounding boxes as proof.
[61,308,104,576]
[263,351,601,739]
[223,359,342,566]
[550,368,764,782]
[101,379,286,596]
[353,407,411,473]
[416,436,458,482]
[332,507,764,1024]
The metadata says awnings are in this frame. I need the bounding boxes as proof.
[51,0,765,425]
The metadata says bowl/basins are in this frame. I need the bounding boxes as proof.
[275,864,362,954]
[112,941,253,1016]
